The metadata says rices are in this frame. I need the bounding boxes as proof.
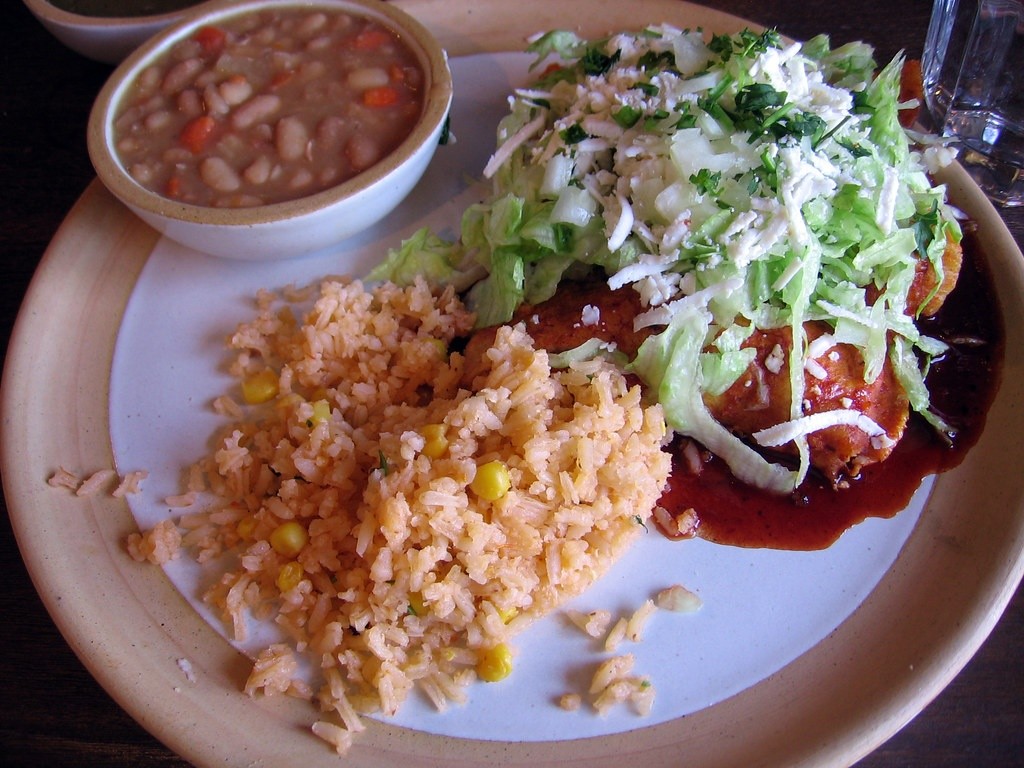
[48,272,705,756]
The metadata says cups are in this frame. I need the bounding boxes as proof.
[916,0,1024,207]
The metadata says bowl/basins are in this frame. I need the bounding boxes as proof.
[23,0,221,65]
[86,0,453,264]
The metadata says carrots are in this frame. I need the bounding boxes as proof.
[161,25,419,204]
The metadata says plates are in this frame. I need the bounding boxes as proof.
[0,0,1024,768]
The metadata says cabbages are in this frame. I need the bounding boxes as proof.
[369,20,969,494]
